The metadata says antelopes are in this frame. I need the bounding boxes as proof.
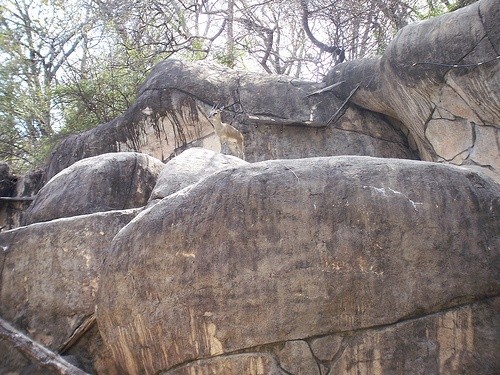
[207,101,245,161]
